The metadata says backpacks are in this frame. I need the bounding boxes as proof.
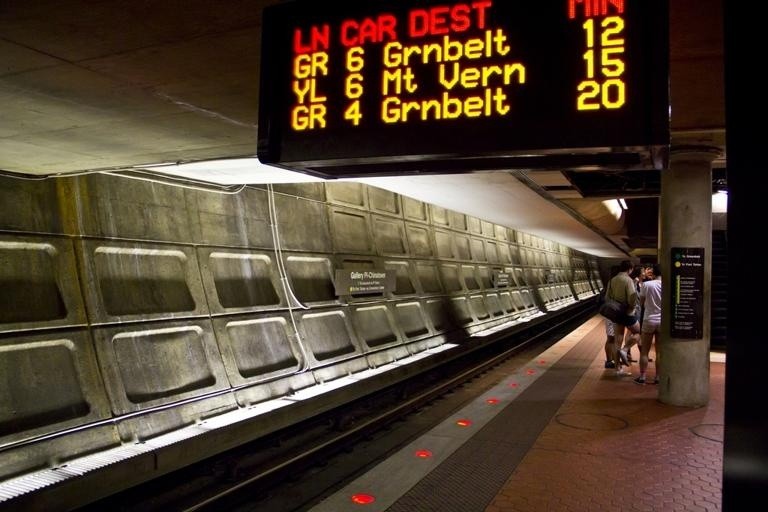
[599,289,606,306]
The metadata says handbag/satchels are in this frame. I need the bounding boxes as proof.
[599,299,625,323]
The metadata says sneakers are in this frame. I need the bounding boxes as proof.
[617,369,632,376]
[654,380,659,385]
[605,350,637,369]
[634,377,645,383]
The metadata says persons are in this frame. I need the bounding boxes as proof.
[598,258,663,385]
